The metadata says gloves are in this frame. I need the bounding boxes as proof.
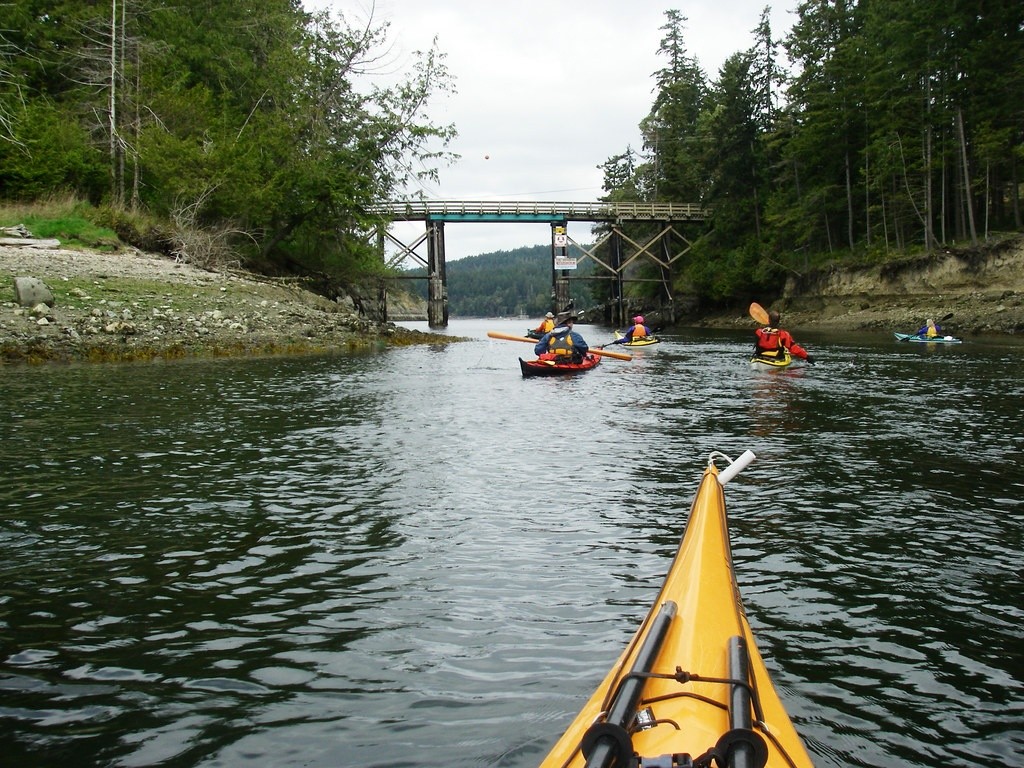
[806,355,815,364]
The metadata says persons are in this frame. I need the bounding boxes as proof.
[918,319,940,339]
[614,316,651,344]
[534,312,588,364]
[753,311,814,364]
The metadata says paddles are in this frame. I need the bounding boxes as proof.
[488,332,632,361]
[524,303,574,339]
[897,313,954,342]
[749,303,816,368]
[593,325,665,350]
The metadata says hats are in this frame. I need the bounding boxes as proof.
[545,312,554,318]
[553,312,578,327]
[632,316,644,323]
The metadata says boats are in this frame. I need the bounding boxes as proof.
[519,344,603,378]
[750,352,792,372]
[894,332,963,345]
[614,330,666,352]
[525,326,547,341]
